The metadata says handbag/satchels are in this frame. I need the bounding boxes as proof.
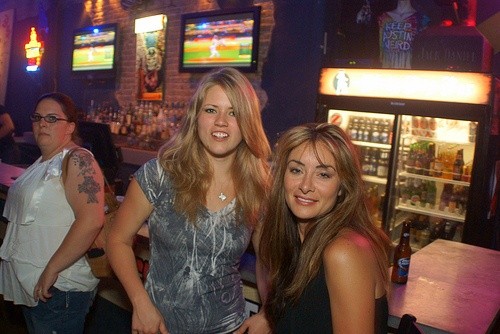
[62,147,136,278]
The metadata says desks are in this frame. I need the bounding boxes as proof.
[113,133,160,165]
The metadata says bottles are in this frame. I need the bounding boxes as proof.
[344,117,472,247]
[86,99,188,139]
[391,222,412,284]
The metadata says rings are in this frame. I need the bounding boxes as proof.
[38,289,43,292]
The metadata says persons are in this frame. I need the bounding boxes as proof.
[0,91,105,334]
[107,67,276,334]
[263,123,392,334]
[210,34,221,57]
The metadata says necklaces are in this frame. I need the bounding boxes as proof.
[211,175,234,203]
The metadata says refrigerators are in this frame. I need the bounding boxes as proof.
[312,59,500,246]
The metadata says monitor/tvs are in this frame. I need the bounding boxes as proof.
[179,5,263,74]
[71,22,118,79]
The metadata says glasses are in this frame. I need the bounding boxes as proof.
[30,115,73,124]
